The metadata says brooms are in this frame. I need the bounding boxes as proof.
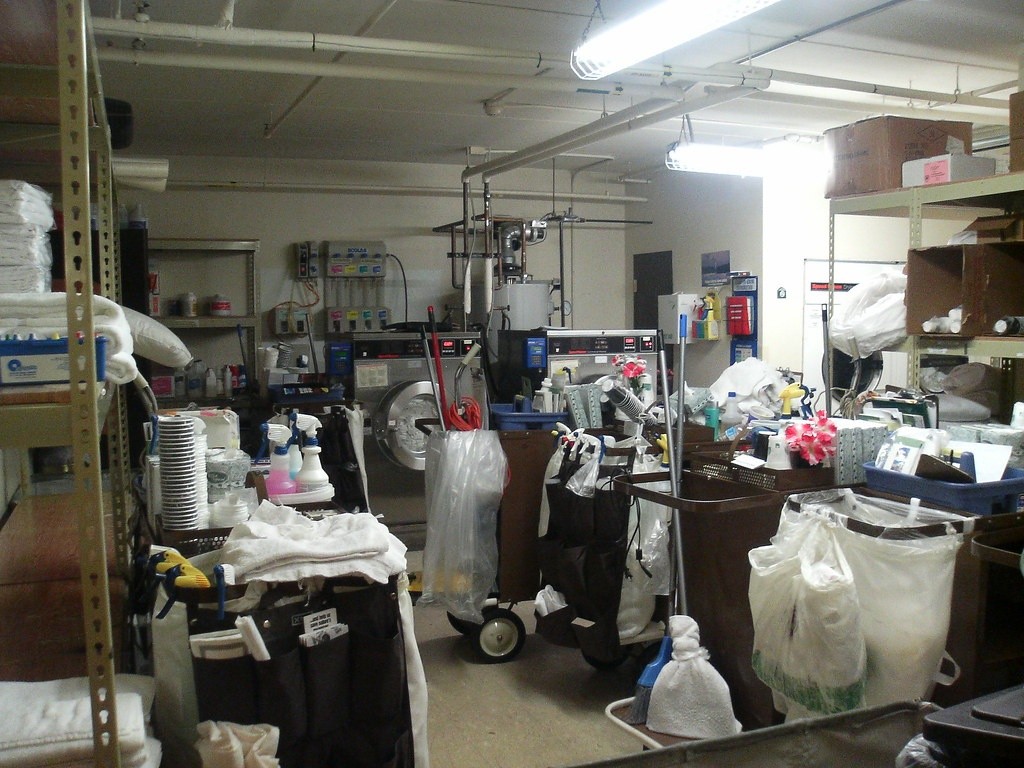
[624,313,745,737]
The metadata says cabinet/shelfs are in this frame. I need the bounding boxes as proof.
[148,241,258,398]
[826,174,1023,419]
[0,0,137,767]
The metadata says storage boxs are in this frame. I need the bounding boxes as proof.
[826,116,976,198]
[862,460,1024,512]
[975,213,1023,243]
[904,244,1023,338]
[901,152,996,186]
[491,403,569,429]
[1009,91,1024,174]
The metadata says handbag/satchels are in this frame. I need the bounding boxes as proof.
[747,516,867,728]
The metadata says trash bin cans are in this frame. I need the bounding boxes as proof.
[903,683,1024,768]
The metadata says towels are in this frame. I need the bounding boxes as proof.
[0,177,139,384]
[534,583,569,619]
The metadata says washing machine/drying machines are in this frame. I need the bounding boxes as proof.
[353,330,482,529]
[546,328,658,406]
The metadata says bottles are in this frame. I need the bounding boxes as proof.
[705,401,719,440]
[206,369,216,397]
[223,366,233,397]
[184,292,197,317]
[189,361,201,398]
[721,392,742,435]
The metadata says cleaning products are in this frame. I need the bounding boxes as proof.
[254,412,330,494]
[691,295,719,341]
[703,392,742,440]
[176,359,247,398]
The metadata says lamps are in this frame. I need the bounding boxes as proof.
[568,0,781,84]
[665,143,823,179]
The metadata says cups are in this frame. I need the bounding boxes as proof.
[599,381,658,428]
[212,301,231,316]
[748,406,775,426]
[257,342,292,385]
[995,317,1024,334]
[158,418,248,531]
[922,308,961,333]
